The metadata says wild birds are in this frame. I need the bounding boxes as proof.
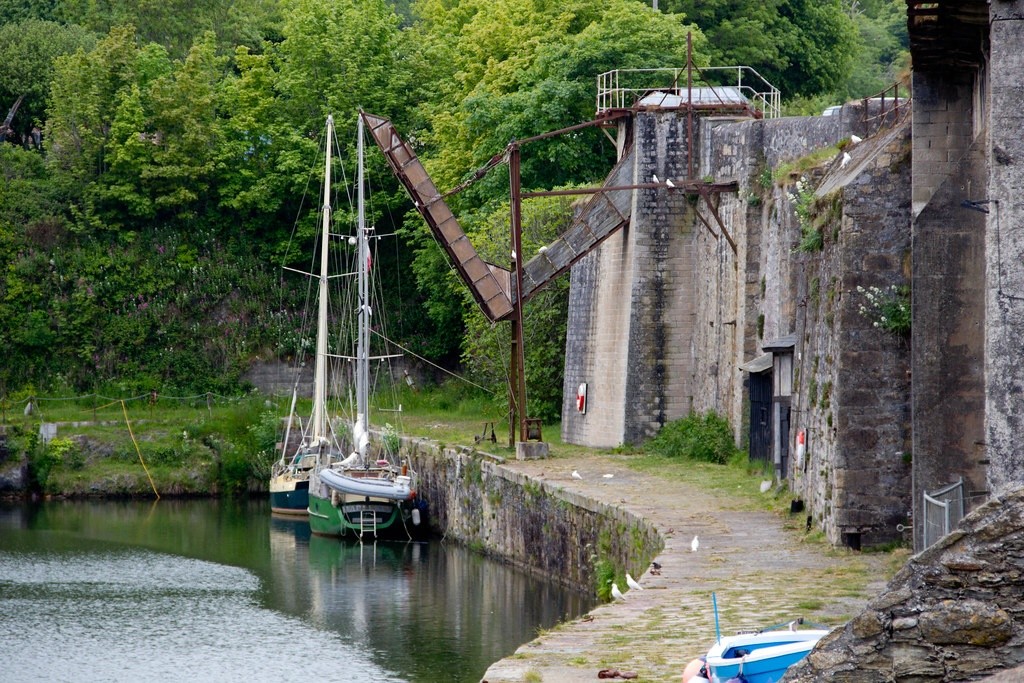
[610,533,700,604]
[651,173,675,188]
[838,132,863,169]
[570,468,615,482]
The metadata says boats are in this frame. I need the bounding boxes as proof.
[681,628,831,682]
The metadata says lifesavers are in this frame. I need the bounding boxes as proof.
[575,381,589,415]
[796,429,807,470]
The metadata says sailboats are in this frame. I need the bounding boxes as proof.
[306,114,427,538]
[269,113,366,516]
